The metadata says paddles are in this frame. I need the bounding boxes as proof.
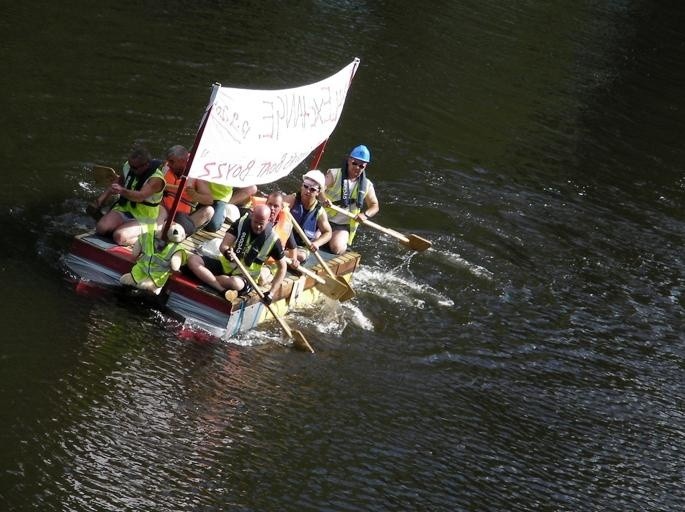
[288,260,347,300]
[95,165,181,192]
[331,204,431,252]
[287,211,356,302]
[231,248,315,354]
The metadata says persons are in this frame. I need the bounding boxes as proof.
[251,191,300,285]
[187,203,287,306]
[95,142,257,247]
[283,171,333,264]
[319,145,380,255]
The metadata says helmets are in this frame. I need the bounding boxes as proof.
[350,145,370,163]
[303,169,325,190]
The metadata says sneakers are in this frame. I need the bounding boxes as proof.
[223,289,238,303]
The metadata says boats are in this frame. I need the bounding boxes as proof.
[57,190,362,342]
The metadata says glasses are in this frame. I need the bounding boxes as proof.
[302,184,319,192]
[352,162,365,168]
